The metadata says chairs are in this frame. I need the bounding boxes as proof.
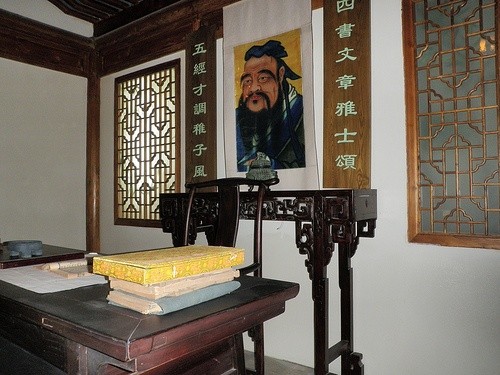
[183,178,279,375]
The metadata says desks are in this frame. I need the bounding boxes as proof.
[0,245,299,375]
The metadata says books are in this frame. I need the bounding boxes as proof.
[37,258,99,278]
[92,245,245,316]
